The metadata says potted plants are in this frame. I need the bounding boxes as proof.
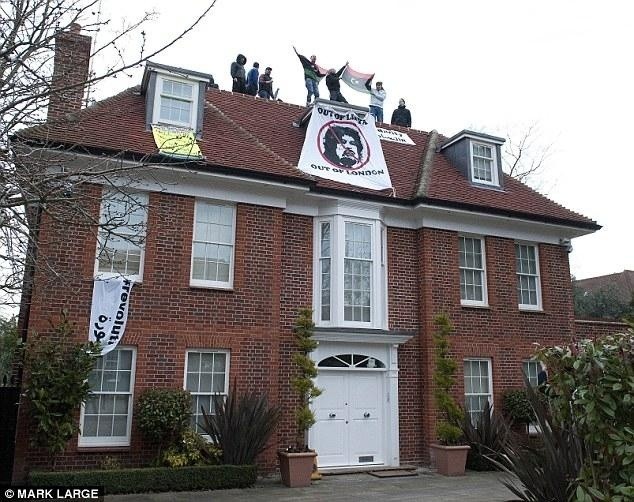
[276,305,324,487]
[429,311,470,476]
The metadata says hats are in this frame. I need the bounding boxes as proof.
[265,66,272,70]
[328,68,336,74]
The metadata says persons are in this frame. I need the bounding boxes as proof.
[321,126,364,168]
[364,73,386,122]
[258,66,274,100]
[230,54,247,93]
[246,62,259,96]
[293,45,327,105]
[391,97,411,128]
[273,87,283,101]
[326,60,351,103]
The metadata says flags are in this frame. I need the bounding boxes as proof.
[342,64,372,94]
[296,104,397,197]
